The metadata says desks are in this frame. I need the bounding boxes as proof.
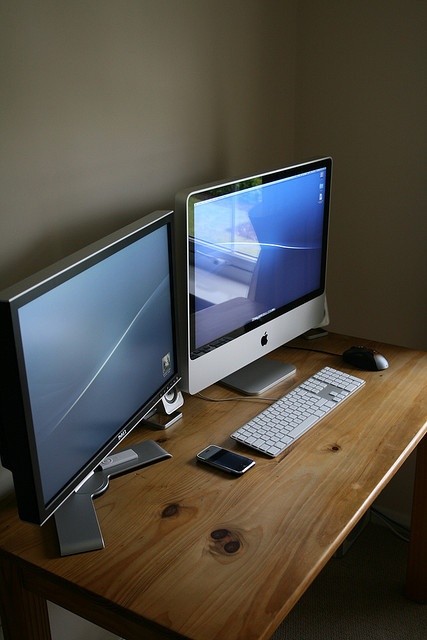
[0,329,427,639]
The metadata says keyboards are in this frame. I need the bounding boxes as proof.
[231,366,367,457]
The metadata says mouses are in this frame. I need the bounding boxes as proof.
[344,344,396,372]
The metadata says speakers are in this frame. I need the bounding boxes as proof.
[161,386,188,416]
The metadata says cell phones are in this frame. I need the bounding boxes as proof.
[196,444,255,477]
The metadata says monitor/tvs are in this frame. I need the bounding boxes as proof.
[0,208,182,559]
[175,156,333,395]
